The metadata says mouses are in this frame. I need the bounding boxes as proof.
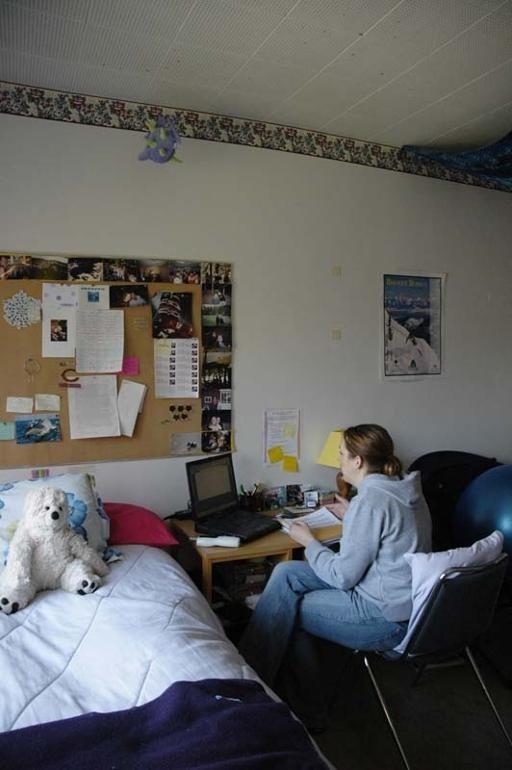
[282,512,296,518]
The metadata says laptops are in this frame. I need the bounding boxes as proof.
[186,453,285,544]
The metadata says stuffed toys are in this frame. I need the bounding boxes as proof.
[0,487,110,616]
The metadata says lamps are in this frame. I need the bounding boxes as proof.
[317,431,354,500]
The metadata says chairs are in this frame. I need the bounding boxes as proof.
[325,553,511,770]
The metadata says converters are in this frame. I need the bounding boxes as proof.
[175,510,193,520]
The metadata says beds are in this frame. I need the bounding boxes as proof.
[0,545,340,770]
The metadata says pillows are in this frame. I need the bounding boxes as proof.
[0,472,124,564]
[393,530,504,656]
[103,502,181,547]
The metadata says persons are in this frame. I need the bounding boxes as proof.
[202,414,231,454]
[166,269,200,285]
[111,289,147,309]
[235,422,433,694]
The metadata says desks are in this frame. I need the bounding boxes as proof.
[169,499,342,611]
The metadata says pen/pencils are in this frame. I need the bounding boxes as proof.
[240,483,259,496]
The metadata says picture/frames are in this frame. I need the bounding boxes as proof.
[377,272,445,382]
